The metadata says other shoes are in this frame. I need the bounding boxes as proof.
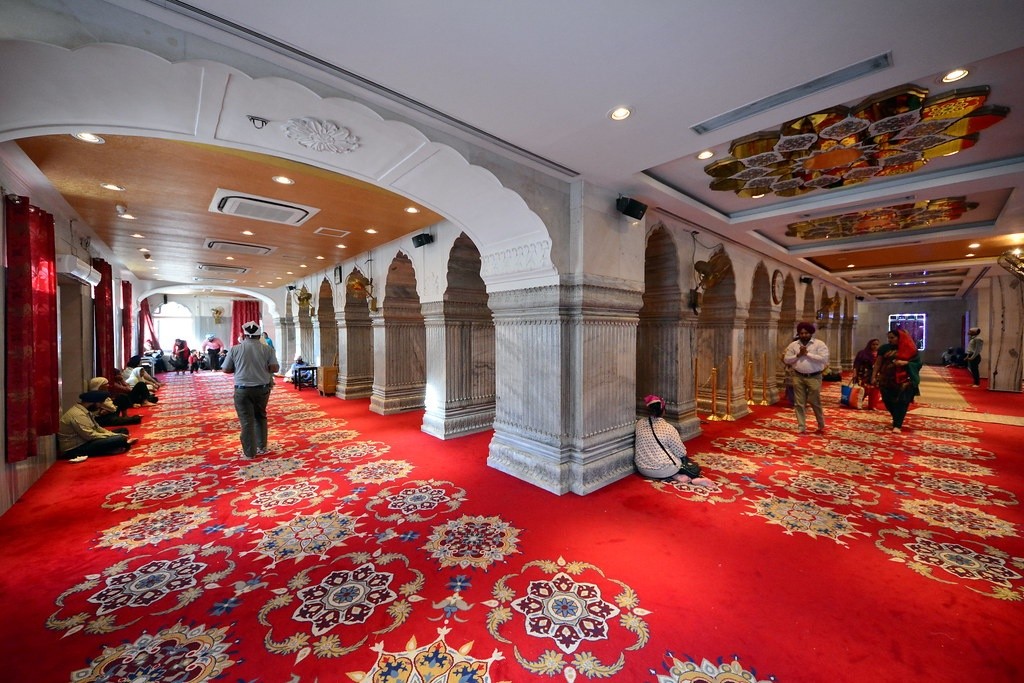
[817,426,824,432]
[893,427,901,434]
[797,427,805,433]
[889,420,895,428]
[971,384,979,388]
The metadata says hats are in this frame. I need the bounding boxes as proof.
[796,323,815,335]
[79,392,108,403]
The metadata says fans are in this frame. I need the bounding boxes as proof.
[687,230,732,308]
[211,306,225,324]
[293,289,315,316]
[817,296,842,319]
[996,251,1024,282]
[345,271,377,311]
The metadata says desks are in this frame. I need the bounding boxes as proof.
[295,366,317,391]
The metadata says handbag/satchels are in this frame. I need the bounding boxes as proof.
[841,377,864,409]
[680,456,701,479]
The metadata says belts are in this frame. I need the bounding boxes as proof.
[233,383,267,389]
[795,370,820,377]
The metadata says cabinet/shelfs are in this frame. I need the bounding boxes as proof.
[317,366,339,396]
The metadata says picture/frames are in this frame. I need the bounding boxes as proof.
[334,266,341,284]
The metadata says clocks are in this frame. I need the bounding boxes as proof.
[771,269,785,304]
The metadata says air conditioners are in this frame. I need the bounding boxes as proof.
[57,254,102,286]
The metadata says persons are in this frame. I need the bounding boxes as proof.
[964,328,983,387]
[941,347,966,367]
[221,321,280,459]
[89,377,141,426]
[871,329,923,434]
[144,340,167,372]
[634,395,714,487]
[202,334,224,371]
[852,339,880,410]
[784,322,829,433]
[291,355,312,383]
[114,355,166,407]
[172,339,190,376]
[262,331,272,348]
[781,338,799,405]
[189,349,203,374]
[58,391,138,457]
[219,350,228,366]
